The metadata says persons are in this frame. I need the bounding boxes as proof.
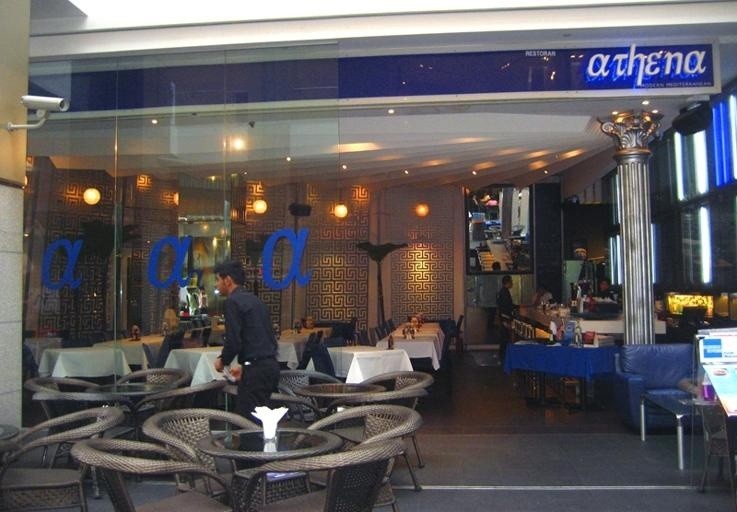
[497,276,517,355]
[600,280,614,298]
[212,262,280,421]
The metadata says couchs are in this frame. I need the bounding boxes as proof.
[612,343,701,432]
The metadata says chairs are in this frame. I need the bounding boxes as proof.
[296,332,316,370]
[388,319,395,332]
[359,371,434,391]
[269,393,324,424]
[698,404,734,494]
[142,408,263,501]
[69,438,236,512]
[156,331,185,368]
[442,314,464,358]
[375,327,384,339]
[189,330,201,347]
[306,405,425,512]
[325,388,429,422]
[22,377,100,464]
[369,328,380,345]
[512,319,553,408]
[245,439,408,512]
[117,368,191,387]
[344,317,357,339]
[275,370,343,412]
[0,406,126,512]
[500,313,518,372]
[132,379,229,459]
[681,306,708,335]
[312,330,323,357]
[201,327,212,347]
[33,393,142,499]
[384,320,390,335]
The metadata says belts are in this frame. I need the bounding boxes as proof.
[241,355,273,363]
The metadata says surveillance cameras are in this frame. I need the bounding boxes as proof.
[6,95,68,130]
[673,99,714,138]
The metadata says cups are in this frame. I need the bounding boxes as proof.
[263,422,277,439]
[702,380,714,401]
